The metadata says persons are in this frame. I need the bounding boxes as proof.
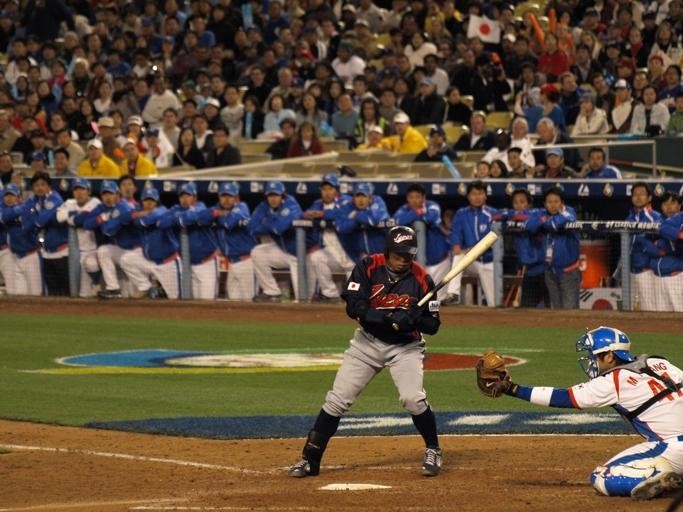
[289,225,444,476]
[476,327,683,501]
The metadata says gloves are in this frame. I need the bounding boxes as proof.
[404,298,424,318]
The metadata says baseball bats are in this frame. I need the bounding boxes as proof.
[390,232,499,331]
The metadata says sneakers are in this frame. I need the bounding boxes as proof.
[288,455,321,479]
[312,292,342,304]
[96,287,123,299]
[437,291,462,306]
[251,290,283,304]
[625,457,682,503]
[420,446,443,477]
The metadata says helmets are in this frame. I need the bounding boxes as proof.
[583,325,637,365]
[384,223,419,257]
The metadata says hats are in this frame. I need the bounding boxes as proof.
[140,188,160,202]
[610,77,631,91]
[544,146,565,158]
[264,180,286,196]
[352,180,374,197]
[97,180,122,195]
[319,173,341,187]
[390,112,412,125]
[417,78,435,88]
[216,182,240,197]
[175,182,199,197]
[28,96,223,164]
[0,182,23,198]
[70,176,91,192]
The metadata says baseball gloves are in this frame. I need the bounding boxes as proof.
[475,350,510,396]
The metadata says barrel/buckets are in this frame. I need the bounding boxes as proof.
[579,240,613,290]
